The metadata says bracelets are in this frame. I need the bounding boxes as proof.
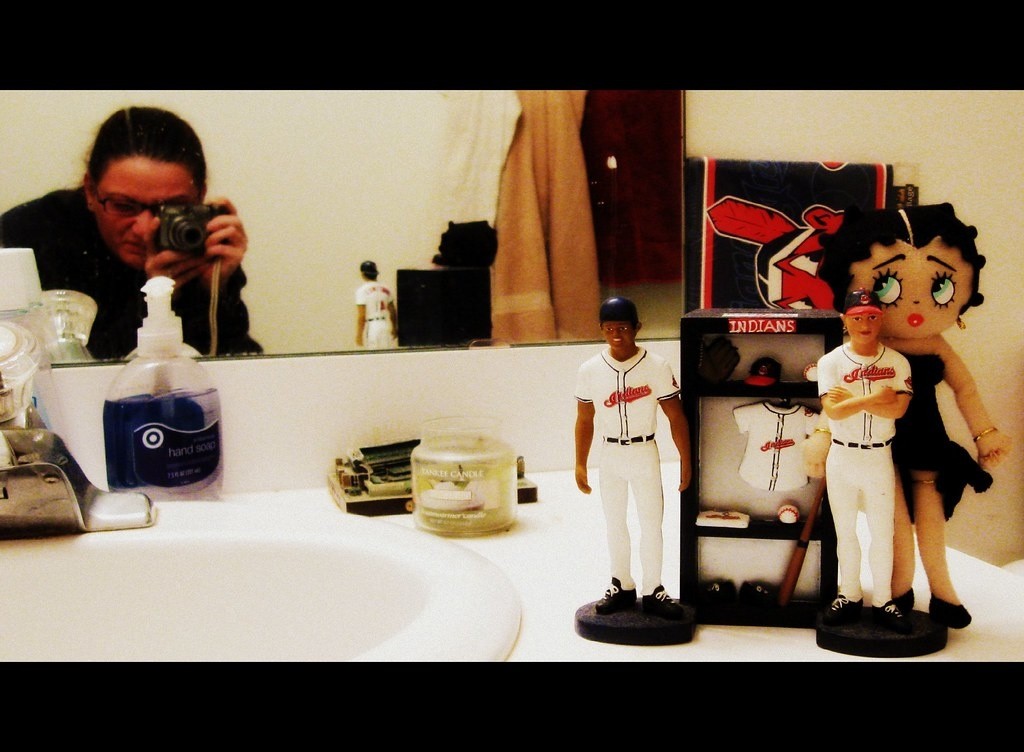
[973,427,997,442]
[812,429,831,436]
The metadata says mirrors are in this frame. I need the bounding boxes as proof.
[0,88,688,367]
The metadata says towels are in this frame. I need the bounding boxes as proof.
[441,90,524,230]
[489,90,607,346]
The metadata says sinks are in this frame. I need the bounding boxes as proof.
[0,506,523,663]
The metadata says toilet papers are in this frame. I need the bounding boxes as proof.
[0,246,43,318]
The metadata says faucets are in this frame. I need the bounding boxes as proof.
[0,317,96,540]
[23,289,99,368]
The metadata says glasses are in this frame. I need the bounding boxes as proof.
[87,186,160,217]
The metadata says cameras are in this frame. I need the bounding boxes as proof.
[153,204,229,259]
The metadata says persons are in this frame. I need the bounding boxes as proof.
[355,261,397,350]
[574,296,692,615]
[0,106,263,360]
[816,290,913,627]
[802,202,1012,630]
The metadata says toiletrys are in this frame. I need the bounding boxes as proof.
[102,275,228,504]
[410,415,519,535]
